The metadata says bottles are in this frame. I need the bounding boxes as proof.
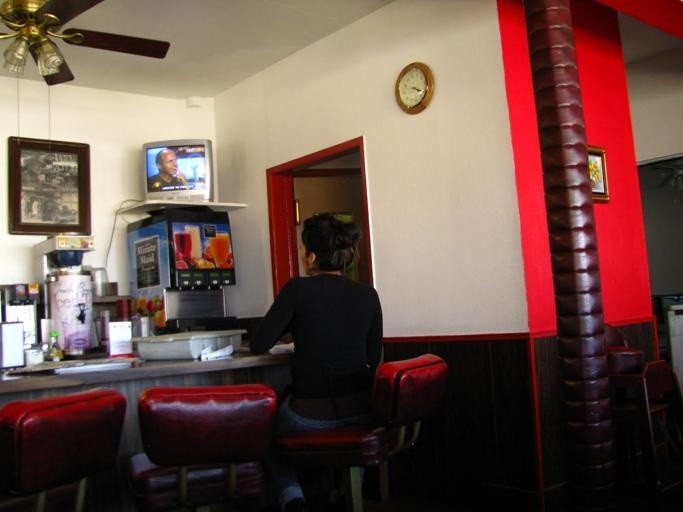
[99,310,109,345]
[46,330,64,362]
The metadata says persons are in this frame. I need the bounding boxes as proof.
[145,148,191,192]
[245,209,385,512]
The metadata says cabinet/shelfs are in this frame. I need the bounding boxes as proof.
[605,354,683,498]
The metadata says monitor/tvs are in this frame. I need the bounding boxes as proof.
[142,139,214,202]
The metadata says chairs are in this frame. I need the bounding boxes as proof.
[1,387,129,512]
[267,351,450,511]
[134,383,278,510]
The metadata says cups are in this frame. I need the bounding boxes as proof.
[23,349,43,367]
[91,266,109,298]
[131,316,148,337]
[173,230,192,260]
[183,224,201,260]
[115,298,132,320]
[206,230,231,268]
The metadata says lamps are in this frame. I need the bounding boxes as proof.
[1,4,85,78]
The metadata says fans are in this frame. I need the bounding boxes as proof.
[1,1,171,88]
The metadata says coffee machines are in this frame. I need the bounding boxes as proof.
[44,231,94,358]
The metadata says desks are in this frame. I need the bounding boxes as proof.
[1,341,294,481]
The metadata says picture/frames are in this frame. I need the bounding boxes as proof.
[584,145,609,204]
[7,135,91,238]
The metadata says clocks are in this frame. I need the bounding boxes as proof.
[392,62,436,116]
